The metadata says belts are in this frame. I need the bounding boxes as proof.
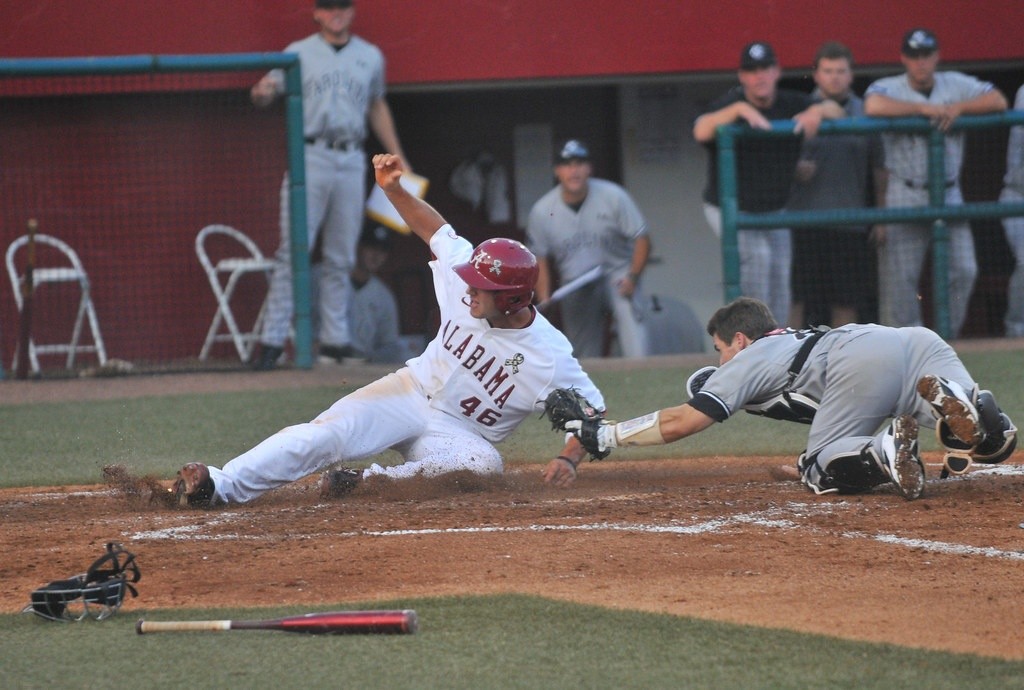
[904,178,955,191]
[304,134,364,151]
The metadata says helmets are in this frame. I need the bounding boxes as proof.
[451,238,540,316]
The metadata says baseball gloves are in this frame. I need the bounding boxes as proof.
[546,388,610,461]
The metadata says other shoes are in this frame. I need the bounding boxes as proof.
[321,342,371,366]
[253,342,287,369]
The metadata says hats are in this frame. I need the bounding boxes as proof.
[739,40,778,69]
[899,30,938,58]
[551,138,591,164]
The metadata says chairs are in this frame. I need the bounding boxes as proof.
[5,233,107,378]
[194,225,296,363]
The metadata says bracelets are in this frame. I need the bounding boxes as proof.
[558,456,576,469]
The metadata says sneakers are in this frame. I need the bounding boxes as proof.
[174,462,213,506]
[883,415,924,501]
[916,376,983,446]
[319,466,359,499]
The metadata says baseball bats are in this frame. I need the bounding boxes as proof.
[137,609,419,634]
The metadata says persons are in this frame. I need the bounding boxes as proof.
[865,28,1006,337]
[998,83,1024,337]
[310,226,408,363]
[794,44,886,331]
[565,297,1017,499]
[175,154,606,506]
[691,40,847,332]
[523,137,652,356]
[249,0,409,371]
